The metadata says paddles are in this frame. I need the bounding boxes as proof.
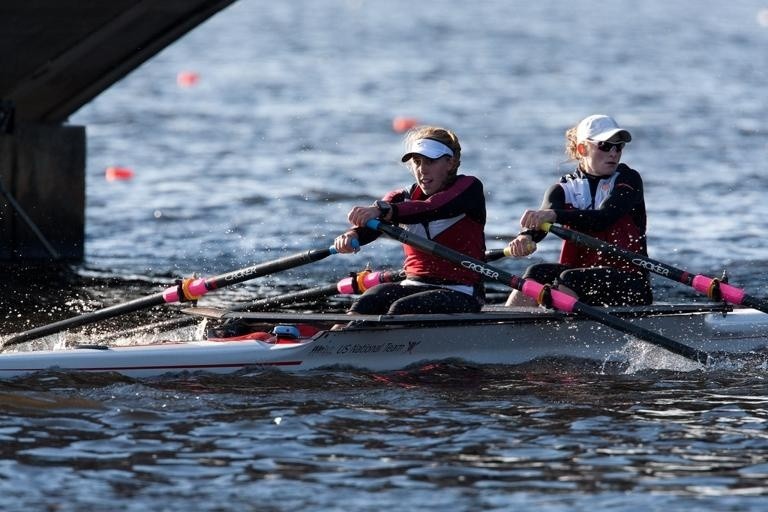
[366,217,763,372]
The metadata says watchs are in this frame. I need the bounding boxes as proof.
[372,200,392,222]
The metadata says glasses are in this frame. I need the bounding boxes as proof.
[598,141,626,152]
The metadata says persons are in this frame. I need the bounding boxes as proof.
[328,125,487,332]
[503,113,654,307]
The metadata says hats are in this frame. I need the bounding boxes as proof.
[577,115,632,143]
[401,138,454,163]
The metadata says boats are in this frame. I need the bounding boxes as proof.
[0,299,767,385]
